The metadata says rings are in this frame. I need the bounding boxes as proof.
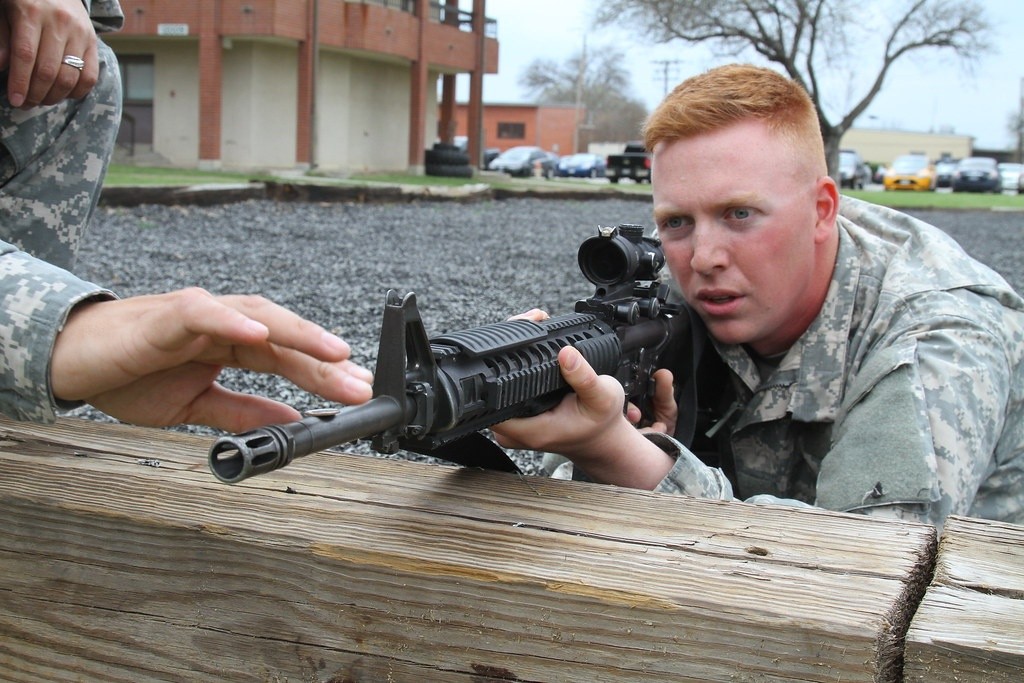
[61,52,82,71]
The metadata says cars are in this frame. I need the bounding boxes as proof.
[951,156,1003,195]
[883,155,936,193]
[874,164,886,184]
[997,161,1024,195]
[487,146,560,178]
[546,150,606,178]
[928,158,955,187]
[839,149,873,191]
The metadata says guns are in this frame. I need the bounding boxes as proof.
[208,222,715,485]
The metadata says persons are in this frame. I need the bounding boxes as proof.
[0,0,374,436]
[486,64,1024,541]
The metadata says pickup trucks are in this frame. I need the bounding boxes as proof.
[453,135,500,165]
[604,144,651,184]
